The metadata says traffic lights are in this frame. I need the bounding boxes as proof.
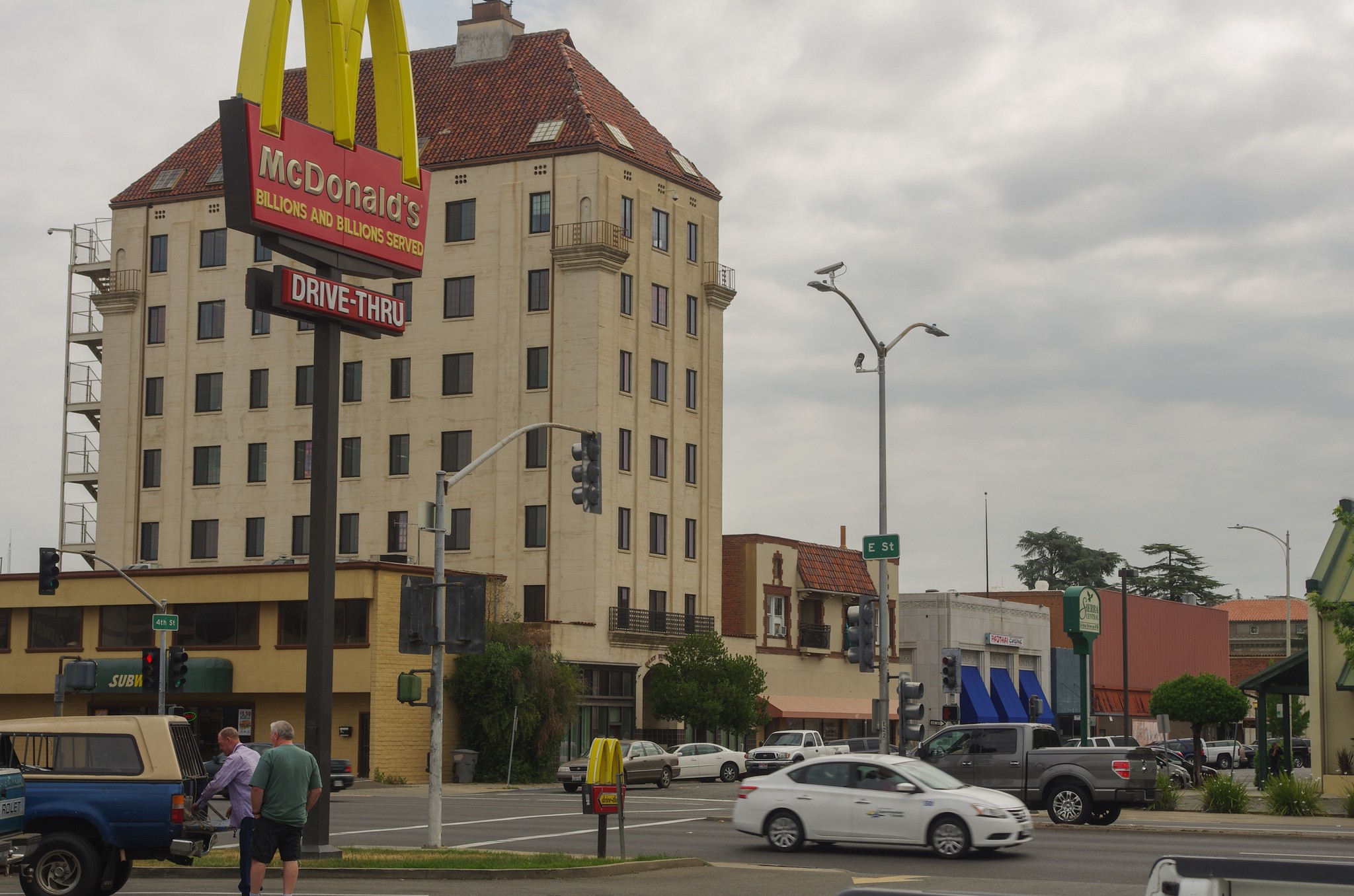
[169,647,189,689]
[942,705,960,721]
[142,648,160,690]
[943,655,957,688]
[572,429,603,514]
[847,596,875,673]
[39,547,59,595]
[896,670,926,742]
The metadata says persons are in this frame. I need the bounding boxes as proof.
[876,772,899,792]
[248,720,323,896]
[191,727,263,896]
[950,734,971,754]
[1161,753,1168,760]
[1269,742,1284,779]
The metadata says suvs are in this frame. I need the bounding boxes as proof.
[1145,738,1311,790]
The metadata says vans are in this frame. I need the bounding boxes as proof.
[0,714,229,896]
[823,737,880,754]
[1063,736,1141,748]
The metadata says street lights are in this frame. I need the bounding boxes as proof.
[1228,524,1290,657]
[808,281,949,750]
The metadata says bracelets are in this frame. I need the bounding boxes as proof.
[252,812,261,815]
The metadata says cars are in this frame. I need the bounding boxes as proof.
[664,743,747,782]
[203,742,353,798]
[732,754,1034,859]
[556,740,680,792]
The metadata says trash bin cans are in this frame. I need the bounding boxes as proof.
[451,749,480,784]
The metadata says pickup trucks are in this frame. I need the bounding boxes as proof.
[745,729,849,776]
[895,722,1157,825]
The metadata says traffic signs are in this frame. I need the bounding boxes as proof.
[863,534,901,558]
